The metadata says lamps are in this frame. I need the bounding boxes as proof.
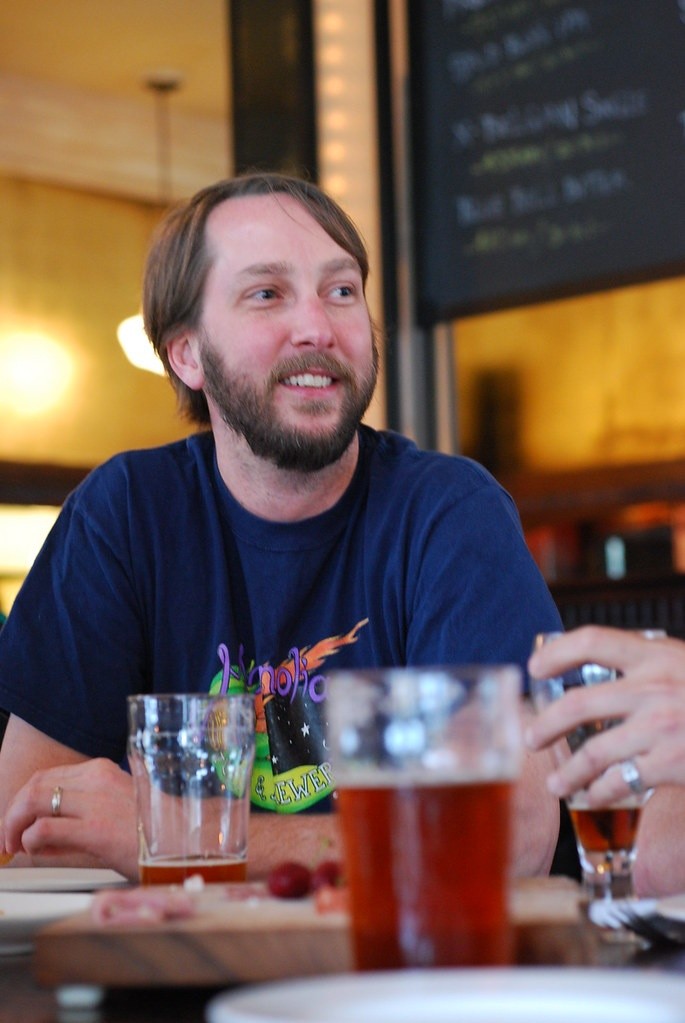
[116,81,169,380]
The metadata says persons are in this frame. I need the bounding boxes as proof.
[0,172,587,890]
[523,618,685,897]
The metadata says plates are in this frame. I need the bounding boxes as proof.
[1,865,127,955]
[210,965,684,1023]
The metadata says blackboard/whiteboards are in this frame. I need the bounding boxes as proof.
[408,1,685,321]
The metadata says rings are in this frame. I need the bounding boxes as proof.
[619,758,644,793]
[51,785,62,816]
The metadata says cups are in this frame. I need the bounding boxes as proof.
[320,662,518,972]
[529,627,668,904]
[128,694,259,884]
